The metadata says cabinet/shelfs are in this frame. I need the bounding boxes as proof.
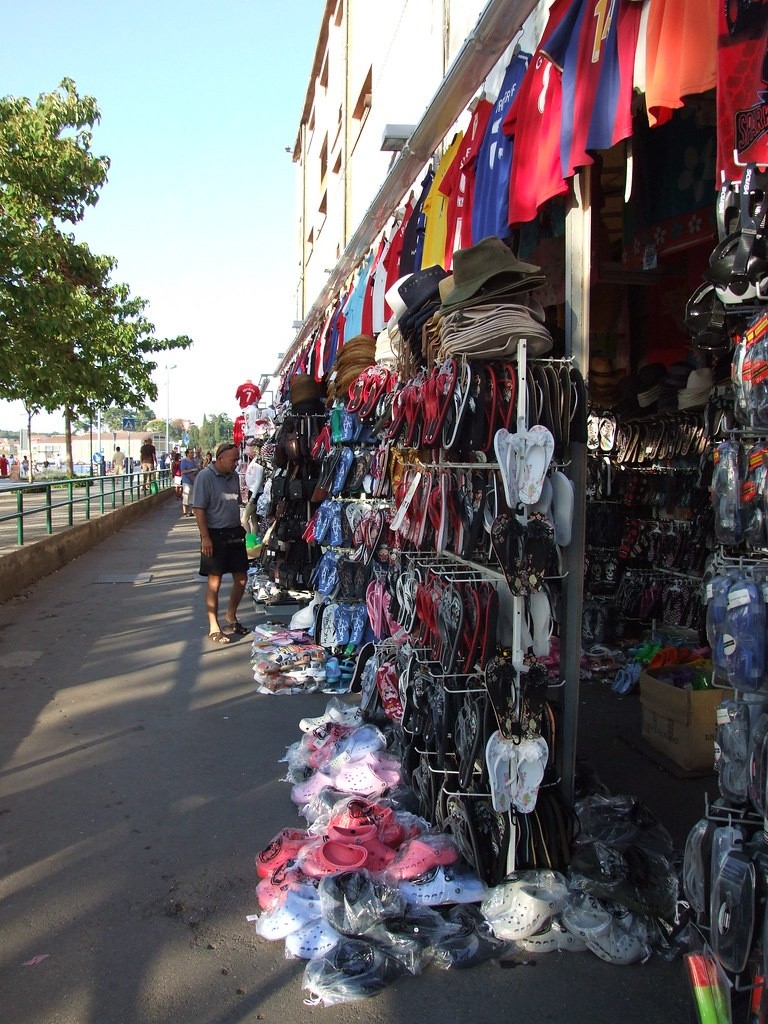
[259,147,768,1024]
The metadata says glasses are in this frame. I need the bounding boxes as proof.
[216,444,238,459]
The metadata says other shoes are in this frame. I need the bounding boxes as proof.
[183,513,194,517]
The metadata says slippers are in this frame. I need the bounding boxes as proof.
[253,162,768,995]
[223,622,247,635]
[208,630,230,644]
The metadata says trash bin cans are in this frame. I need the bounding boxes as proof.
[97,461,106,476]
[125,457,134,474]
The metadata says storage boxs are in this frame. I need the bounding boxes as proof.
[639,668,735,771]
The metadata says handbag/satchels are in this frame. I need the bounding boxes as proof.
[190,475,195,480]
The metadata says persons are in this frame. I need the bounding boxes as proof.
[100,438,215,517]
[192,443,251,643]
[234,380,277,534]
[0,453,63,480]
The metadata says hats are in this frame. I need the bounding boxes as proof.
[589,333,712,410]
[247,438,264,447]
[291,236,552,405]
